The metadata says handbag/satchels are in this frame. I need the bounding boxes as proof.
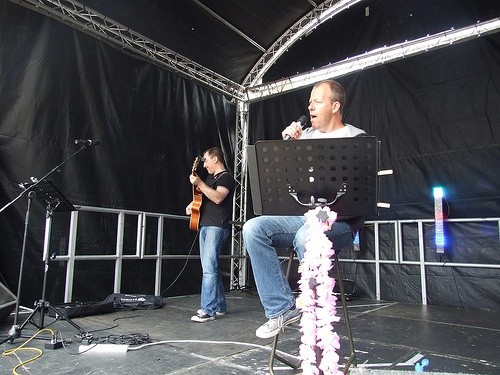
[106,293,164,309]
[49,299,114,319]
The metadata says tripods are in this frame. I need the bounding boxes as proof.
[0,147,91,345]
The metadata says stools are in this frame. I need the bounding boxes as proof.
[269,246,357,375]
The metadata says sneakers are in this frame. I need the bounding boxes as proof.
[215,312,226,315]
[191,309,215,322]
[256,308,301,339]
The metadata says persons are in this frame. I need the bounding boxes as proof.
[186,147,236,322]
[242,80,368,342]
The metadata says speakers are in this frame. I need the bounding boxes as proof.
[0,284,21,323]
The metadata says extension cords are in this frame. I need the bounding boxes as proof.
[79,344,130,355]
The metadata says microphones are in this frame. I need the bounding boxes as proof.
[284,115,309,140]
[75,140,100,146]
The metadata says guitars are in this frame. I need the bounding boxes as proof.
[189,155,205,233]
